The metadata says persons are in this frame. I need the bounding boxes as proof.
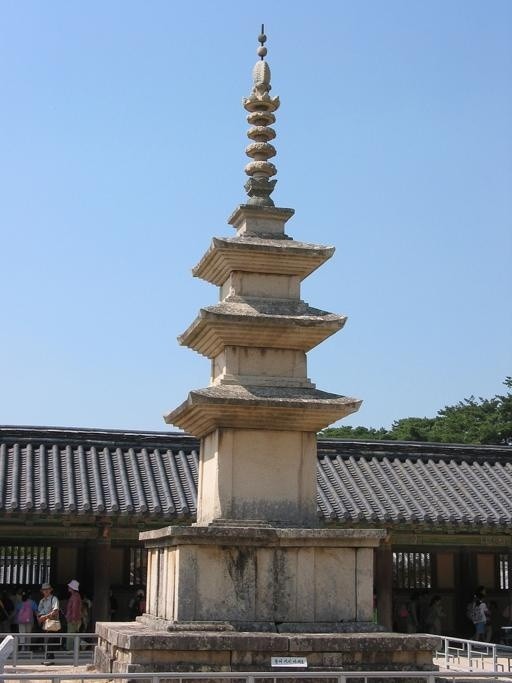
[127,587,139,621]
[373,582,512,649]
[139,587,146,616]
[109,585,118,621]
[0,578,95,665]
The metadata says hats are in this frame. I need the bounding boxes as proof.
[40,582,54,592]
[67,579,80,592]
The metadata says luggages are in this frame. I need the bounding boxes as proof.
[18,599,34,624]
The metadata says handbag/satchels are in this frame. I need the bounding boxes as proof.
[42,617,62,631]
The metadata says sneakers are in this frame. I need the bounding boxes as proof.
[63,640,88,656]
[40,659,54,666]
